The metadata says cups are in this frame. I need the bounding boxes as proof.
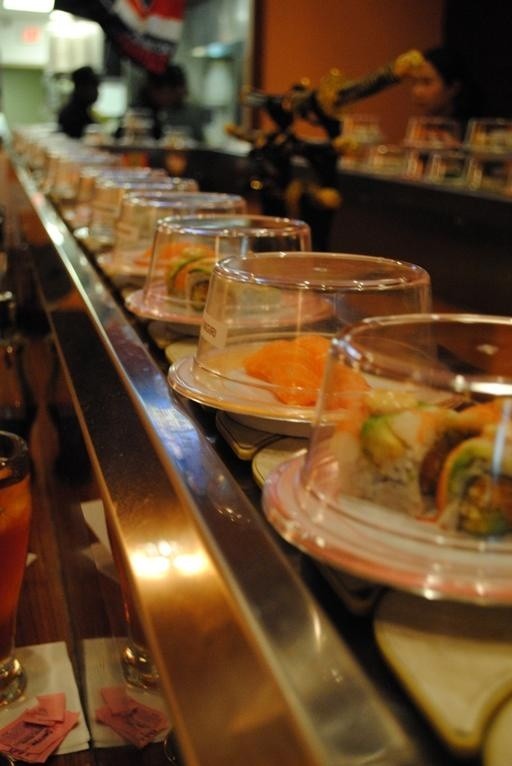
[0,425,33,709]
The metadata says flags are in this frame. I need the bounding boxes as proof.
[53,0,185,73]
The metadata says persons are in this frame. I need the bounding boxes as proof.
[406,46,476,136]
[114,62,205,141]
[58,64,108,135]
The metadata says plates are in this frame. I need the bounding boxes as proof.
[265,437,511,609]
[74,225,223,286]
[167,329,474,440]
[124,276,339,339]
